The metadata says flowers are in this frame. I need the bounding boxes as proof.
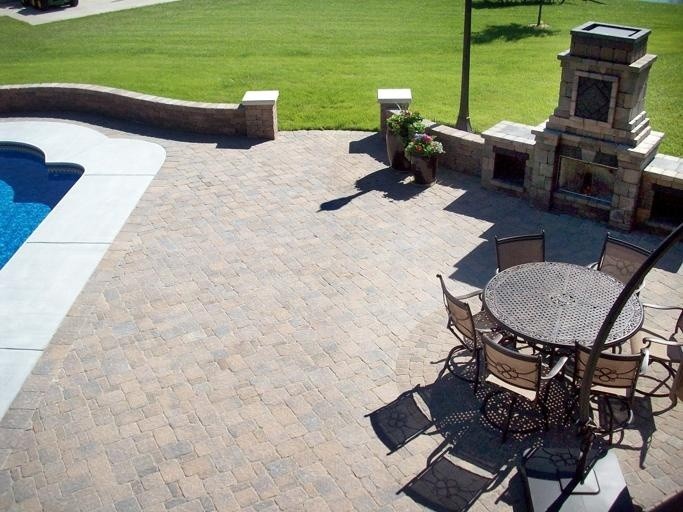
[404,132,446,163]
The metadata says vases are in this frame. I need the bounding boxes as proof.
[411,156,437,188]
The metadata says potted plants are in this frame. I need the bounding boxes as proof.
[383,100,425,173]
